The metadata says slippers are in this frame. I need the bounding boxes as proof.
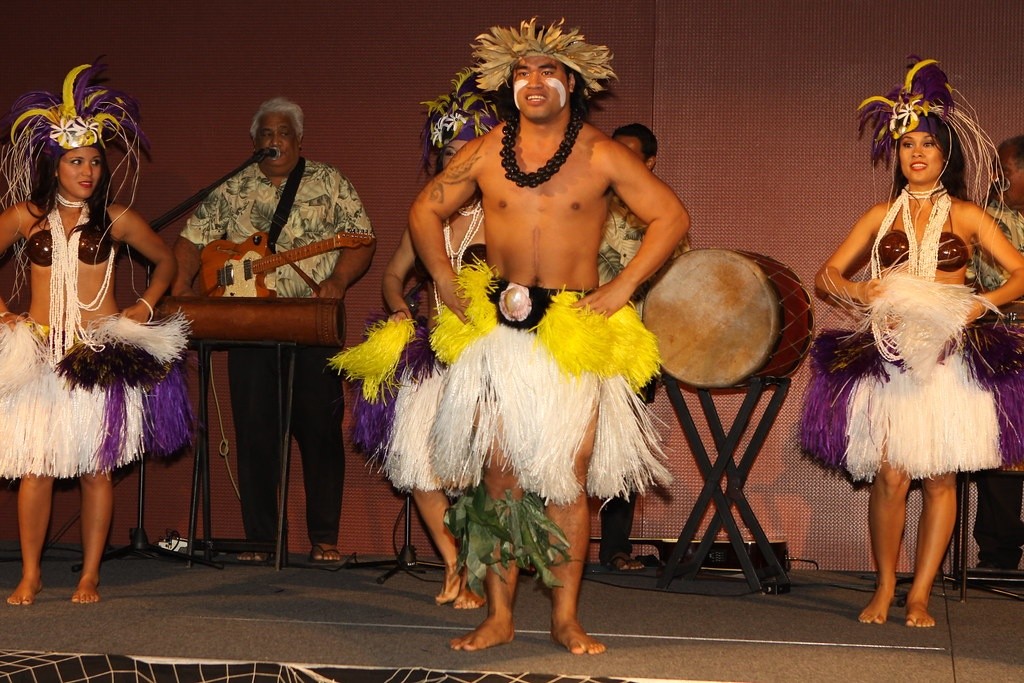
[307,543,340,563]
[605,554,647,575]
[236,544,274,566]
[518,562,541,578]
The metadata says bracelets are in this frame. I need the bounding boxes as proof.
[136,298,154,323]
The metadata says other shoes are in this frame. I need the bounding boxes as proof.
[960,565,1018,589]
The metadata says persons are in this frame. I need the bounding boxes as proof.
[518,122,690,574]
[0,140,176,606]
[382,119,487,611]
[407,26,690,655]
[814,116,1024,629]
[170,95,376,564]
[964,134,1024,584]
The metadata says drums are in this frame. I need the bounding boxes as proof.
[639,245,815,389]
[148,295,346,346]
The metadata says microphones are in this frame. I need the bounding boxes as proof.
[995,177,1011,191]
[258,147,281,160]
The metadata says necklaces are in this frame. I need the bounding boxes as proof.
[500,108,583,187]
[860,180,952,365]
[45,187,116,371]
[433,196,485,315]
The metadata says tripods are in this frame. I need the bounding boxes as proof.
[344,494,447,585]
[70,148,268,572]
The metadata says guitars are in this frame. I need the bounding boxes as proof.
[590,536,790,573]
[200,225,373,298]
